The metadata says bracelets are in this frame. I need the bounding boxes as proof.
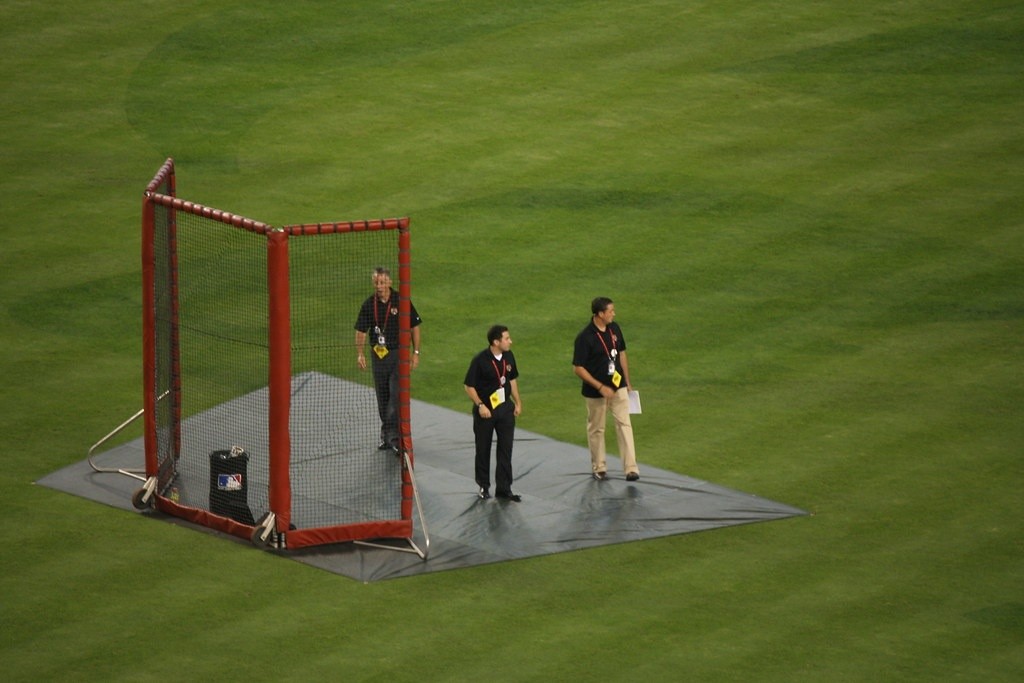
[413,351,420,355]
[598,382,604,392]
[477,402,485,409]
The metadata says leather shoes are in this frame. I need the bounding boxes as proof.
[377,440,388,448]
[392,445,402,454]
[478,488,490,499]
[508,493,520,501]
[627,471,637,481]
[592,471,606,480]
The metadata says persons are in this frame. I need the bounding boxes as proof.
[574,297,641,481]
[463,325,522,501]
[354,267,424,455]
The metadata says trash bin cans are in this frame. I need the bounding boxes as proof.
[208,448,250,515]
